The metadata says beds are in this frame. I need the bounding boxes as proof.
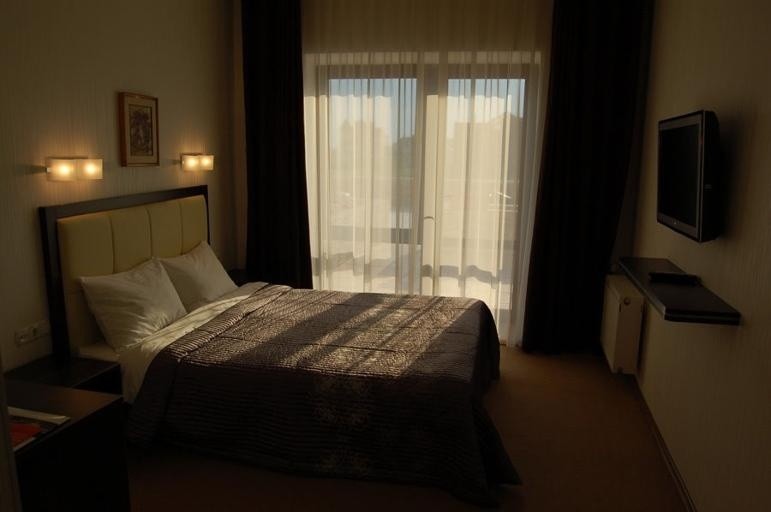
[38,185,494,485]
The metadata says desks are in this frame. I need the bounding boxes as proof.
[0,380,128,512]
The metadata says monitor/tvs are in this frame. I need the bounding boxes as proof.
[656,110,725,243]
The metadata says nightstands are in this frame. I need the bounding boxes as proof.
[4,354,123,394]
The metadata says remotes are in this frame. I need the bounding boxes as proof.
[649,272,697,282]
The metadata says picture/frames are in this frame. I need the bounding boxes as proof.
[119,92,159,167]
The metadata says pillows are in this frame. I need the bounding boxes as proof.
[81,242,238,348]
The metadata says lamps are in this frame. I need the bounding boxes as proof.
[45,156,103,182]
[179,152,214,172]
